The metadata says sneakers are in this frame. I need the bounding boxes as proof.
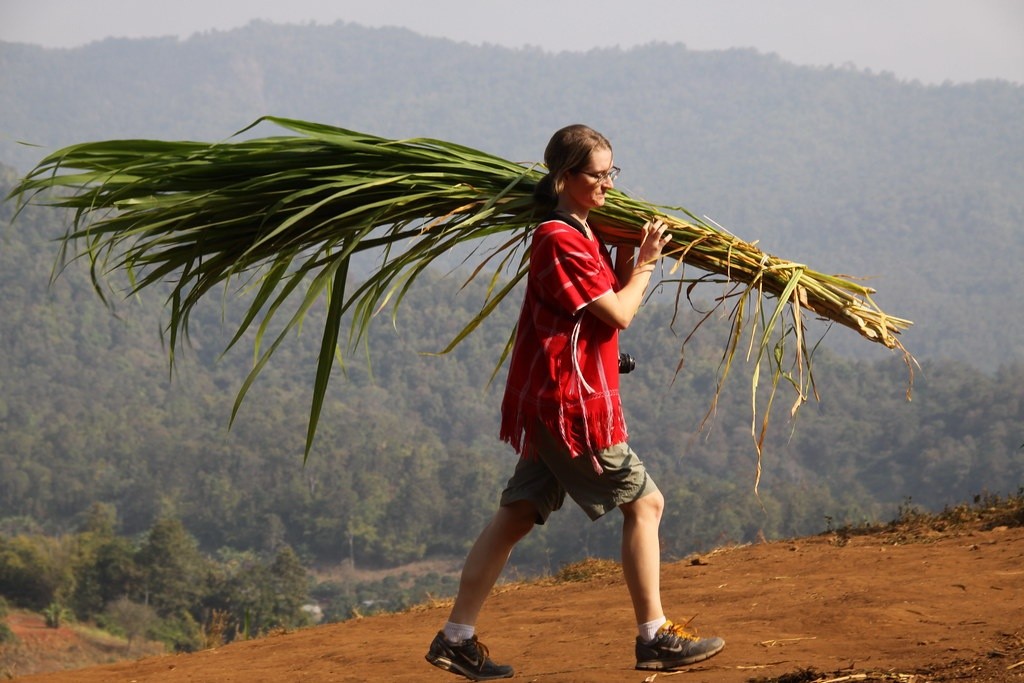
[425,629,513,681]
[635,620,725,669]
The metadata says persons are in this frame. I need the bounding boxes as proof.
[425,125,725,682]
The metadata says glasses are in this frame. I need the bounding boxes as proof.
[580,166,621,183]
